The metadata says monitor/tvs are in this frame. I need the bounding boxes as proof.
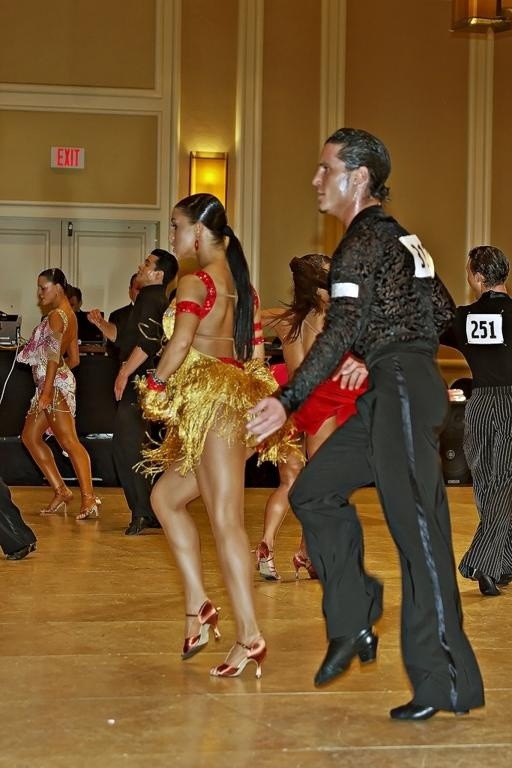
[75,312,104,344]
[0,314,22,346]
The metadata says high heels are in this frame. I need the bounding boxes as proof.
[209,629,268,679]
[313,626,379,687]
[250,539,282,581]
[468,567,511,596]
[180,599,221,660]
[389,698,438,720]
[292,548,320,580]
[4,489,164,561]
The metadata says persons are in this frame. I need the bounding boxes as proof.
[105,273,144,358]
[0,475,37,559]
[256,254,368,583]
[86,248,179,535]
[247,129,485,720]
[134,194,279,679]
[66,287,83,312]
[17,268,102,519]
[437,246,511,596]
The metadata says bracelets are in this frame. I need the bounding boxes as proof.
[147,372,165,391]
